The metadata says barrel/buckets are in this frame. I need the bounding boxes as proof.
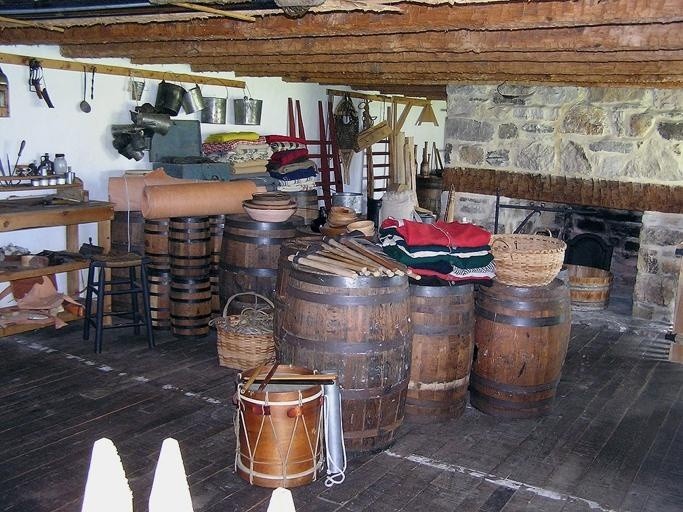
[233,81,263,128]
[402,272,473,429]
[413,177,443,214]
[275,241,412,457]
[175,74,205,115]
[127,73,148,102]
[108,172,365,340]
[564,262,610,315]
[155,72,185,119]
[470,272,573,423]
[198,77,231,125]
[110,100,174,161]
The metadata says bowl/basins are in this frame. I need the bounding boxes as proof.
[243,192,297,224]
[348,220,374,233]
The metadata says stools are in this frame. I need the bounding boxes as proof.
[82,256,155,354]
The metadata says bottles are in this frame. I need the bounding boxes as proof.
[31,153,75,186]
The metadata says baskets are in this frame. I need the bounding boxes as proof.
[214,291,277,372]
[488,227,567,287]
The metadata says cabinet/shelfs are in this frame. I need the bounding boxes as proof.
[0,196,117,336]
